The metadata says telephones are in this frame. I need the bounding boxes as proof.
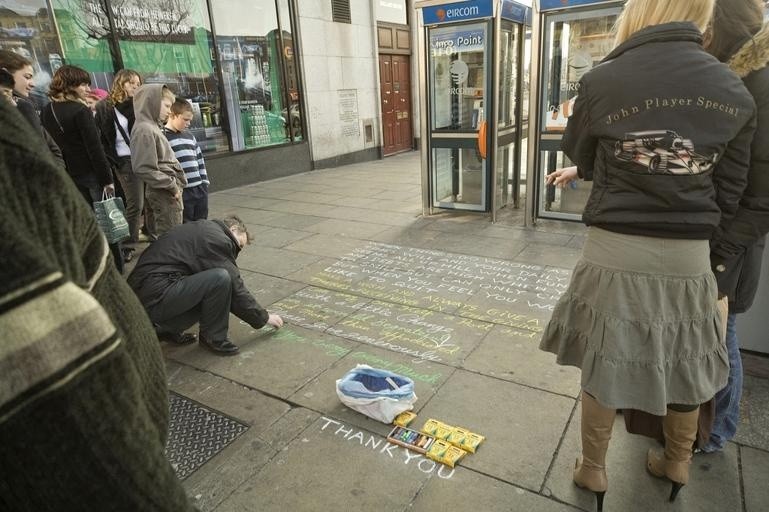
[471,99,483,130]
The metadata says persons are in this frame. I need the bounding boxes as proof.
[544,0,769,454]
[538,0,759,510]
[126,214,282,356]
[1,85,201,512]
[2,48,211,274]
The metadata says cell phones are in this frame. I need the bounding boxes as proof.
[386,424,435,456]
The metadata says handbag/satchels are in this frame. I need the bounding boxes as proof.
[335,363,418,425]
[92,189,131,244]
[624,396,715,448]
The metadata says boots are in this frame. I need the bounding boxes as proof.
[573,390,617,512]
[647,404,700,502]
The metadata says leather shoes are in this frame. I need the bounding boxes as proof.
[121,247,132,261]
[199,339,240,356]
[170,333,197,346]
